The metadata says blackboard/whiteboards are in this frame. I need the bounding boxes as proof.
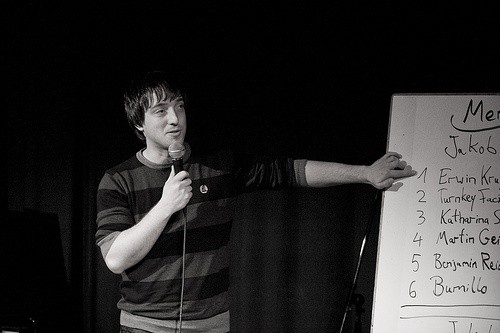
[368,93,500,333]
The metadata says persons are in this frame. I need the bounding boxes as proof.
[94,71,418,333]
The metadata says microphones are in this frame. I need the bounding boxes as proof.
[170,142,187,214]
